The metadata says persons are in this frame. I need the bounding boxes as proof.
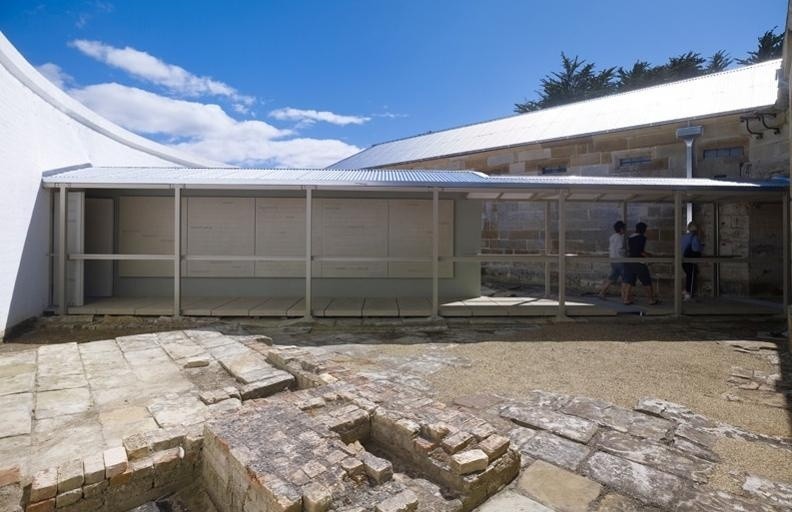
[679,221,704,302]
[596,221,628,301]
[623,222,662,305]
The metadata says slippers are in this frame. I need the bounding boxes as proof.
[648,298,663,306]
[597,293,607,301]
[625,300,635,305]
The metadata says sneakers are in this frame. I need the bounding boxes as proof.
[682,290,687,296]
[685,294,696,303]
